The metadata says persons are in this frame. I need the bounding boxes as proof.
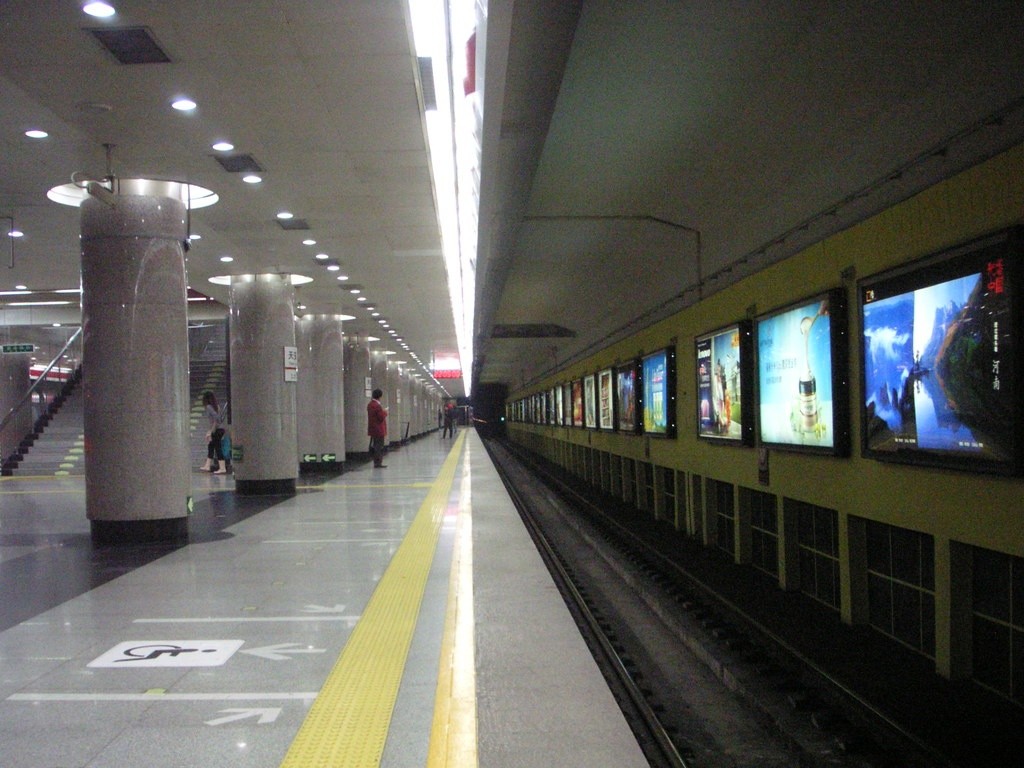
[199,391,227,474]
[469,411,474,427]
[439,405,458,440]
[367,389,388,468]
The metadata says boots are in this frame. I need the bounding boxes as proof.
[213,460,226,474]
[200,458,212,471]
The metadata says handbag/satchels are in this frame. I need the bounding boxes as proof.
[369,446,385,457]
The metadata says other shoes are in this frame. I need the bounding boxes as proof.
[374,464,387,468]
